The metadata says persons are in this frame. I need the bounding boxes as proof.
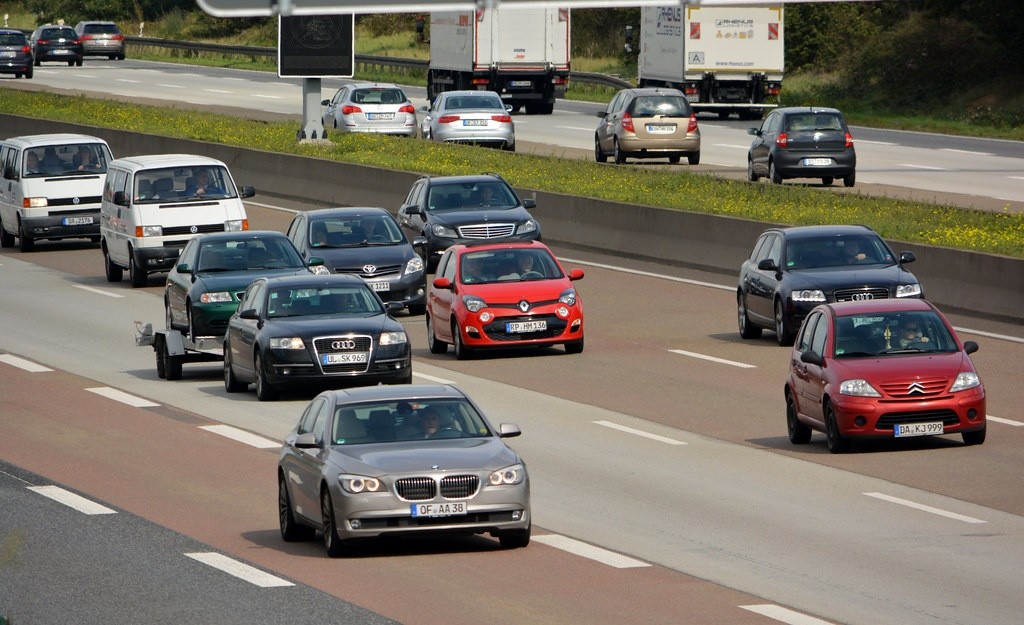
[328,295,355,313]
[410,405,452,438]
[892,317,931,349]
[74,146,101,170]
[38,147,70,170]
[315,227,331,245]
[338,411,371,439]
[477,185,503,204]
[515,253,544,277]
[341,218,392,242]
[185,168,225,196]
[839,241,873,264]
[466,258,496,281]
[27,151,47,174]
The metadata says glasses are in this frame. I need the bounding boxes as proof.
[904,327,917,332]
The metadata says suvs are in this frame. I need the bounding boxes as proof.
[397,169,544,274]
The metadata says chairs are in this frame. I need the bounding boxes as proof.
[338,409,417,445]
[814,116,830,127]
[271,291,333,314]
[139,177,179,197]
[381,92,392,102]
[837,319,900,352]
[202,247,265,270]
[431,190,481,210]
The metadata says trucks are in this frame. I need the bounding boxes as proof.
[621,4,787,119]
[413,5,571,114]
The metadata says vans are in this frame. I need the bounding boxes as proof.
[98,152,257,291]
[0,134,118,252]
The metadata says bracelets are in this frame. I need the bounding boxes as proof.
[196,192,200,195]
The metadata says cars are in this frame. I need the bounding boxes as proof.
[592,85,702,166]
[0,28,36,80]
[163,229,324,344]
[275,382,531,560]
[72,20,126,60]
[780,296,988,454]
[424,236,585,361]
[284,205,431,315]
[733,224,924,347]
[220,273,413,400]
[25,24,86,69]
[320,81,417,138]
[745,106,858,187]
[417,89,518,152]
